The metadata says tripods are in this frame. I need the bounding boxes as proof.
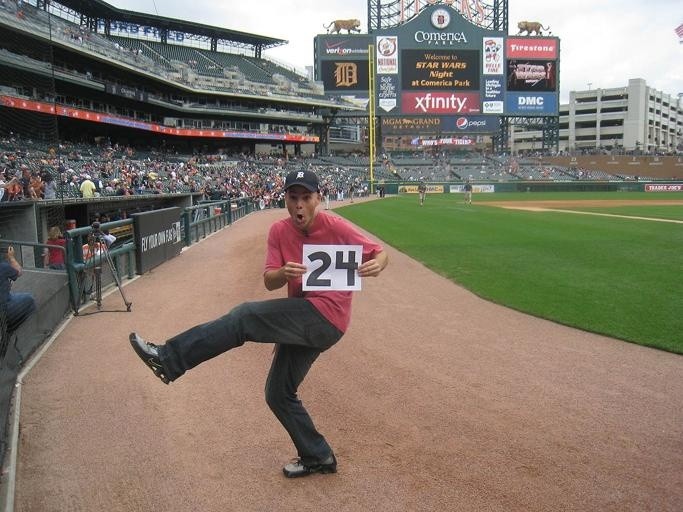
[74,235,131,316]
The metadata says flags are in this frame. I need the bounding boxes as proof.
[675,24,683,40]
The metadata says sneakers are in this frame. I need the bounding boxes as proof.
[283,455,337,478]
[129,333,171,385]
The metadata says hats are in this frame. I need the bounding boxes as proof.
[283,169,320,193]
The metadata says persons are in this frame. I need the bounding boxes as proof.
[13,6,199,70]
[126,170,389,479]
[0,131,385,272]
[0,244,37,357]
[385,147,682,205]
